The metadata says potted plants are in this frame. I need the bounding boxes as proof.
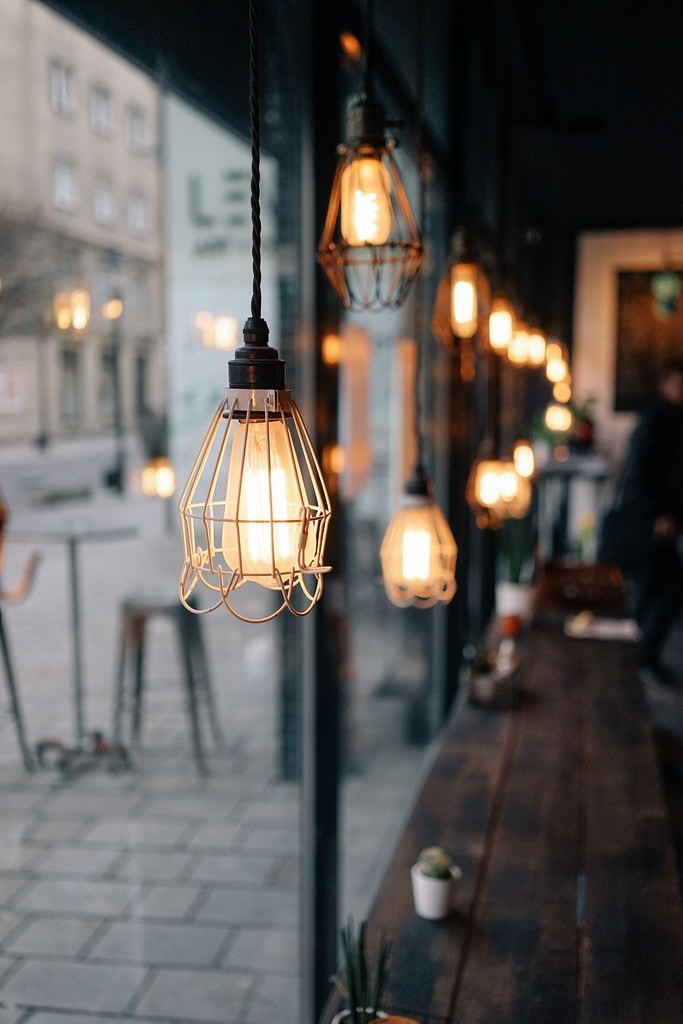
[496,543,538,628]
[409,849,463,920]
[325,914,391,1024]
[565,374,602,442]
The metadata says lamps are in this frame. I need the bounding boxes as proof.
[316,0,423,313]
[379,38,460,608]
[177,0,335,625]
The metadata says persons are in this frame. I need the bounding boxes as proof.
[613,354,683,688]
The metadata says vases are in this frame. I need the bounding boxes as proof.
[501,615,520,636]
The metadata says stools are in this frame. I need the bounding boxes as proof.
[113,587,221,784]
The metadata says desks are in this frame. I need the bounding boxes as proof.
[5,513,141,778]
